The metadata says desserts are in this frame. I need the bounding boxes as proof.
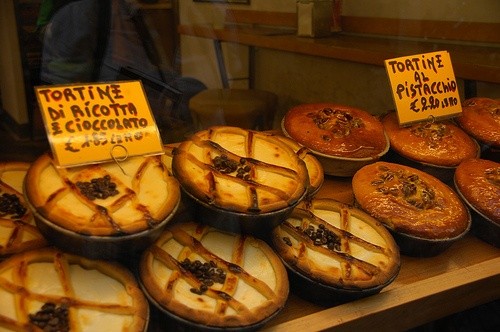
[281,96,500,254]
[0,127,403,332]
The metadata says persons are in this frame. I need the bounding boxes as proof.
[41,0,205,146]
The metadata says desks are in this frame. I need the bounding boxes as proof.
[178,22,500,98]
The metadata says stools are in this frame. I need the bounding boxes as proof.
[188,87,276,131]
